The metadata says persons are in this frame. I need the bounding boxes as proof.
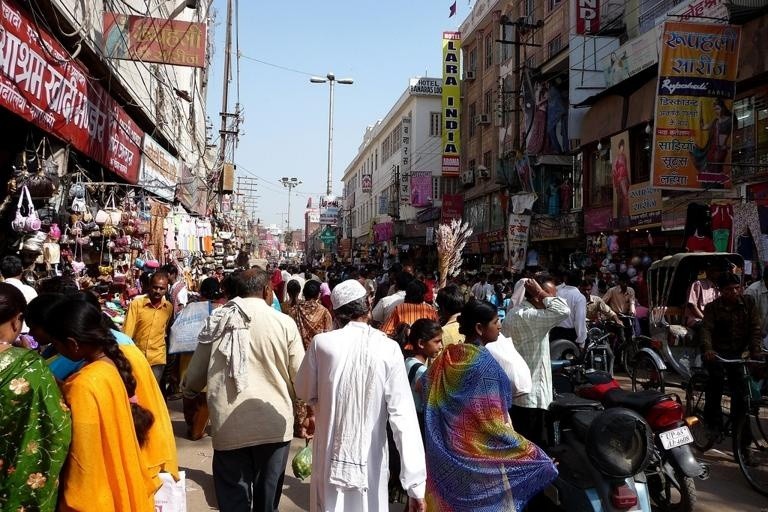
[369,272,413,328]
[543,76,562,153]
[140,272,155,293]
[325,258,357,283]
[198,267,208,290]
[578,280,625,329]
[377,280,439,337]
[374,263,402,305]
[0,254,40,334]
[489,283,507,307]
[743,264,768,354]
[700,274,764,467]
[471,271,490,302]
[404,319,443,451]
[364,269,377,294]
[685,267,721,334]
[0,281,73,512]
[289,280,332,352]
[271,263,283,302]
[295,279,428,512]
[416,298,560,512]
[503,281,515,294]
[123,273,173,385]
[613,140,628,217]
[42,297,180,512]
[432,270,440,282]
[179,268,315,512]
[689,96,734,187]
[160,265,190,307]
[552,268,587,359]
[358,268,366,286]
[281,265,291,302]
[176,276,221,441]
[429,286,466,365]
[26,295,137,382]
[266,265,270,270]
[526,79,548,153]
[501,276,571,454]
[215,266,224,282]
[280,280,305,322]
[600,274,638,316]
[285,268,306,300]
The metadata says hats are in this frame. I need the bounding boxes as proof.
[330,279,367,310]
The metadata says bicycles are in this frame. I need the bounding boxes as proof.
[619,312,667,392]
[685,354,768,496]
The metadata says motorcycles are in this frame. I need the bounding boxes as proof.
[550,332,705,512]
[587,319,617,372]
[545,386,651,512]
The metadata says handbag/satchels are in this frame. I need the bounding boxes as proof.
[72,172,87,212]
[11,186,41,233]
[83,205,93,223]
[95,190,122,225]
[71,228,85,272]
[113,184,153,252]
[98,215,112,274]
[81,218,95,230]
[145,250,160,268]
[101,213,117,237]
[126,287,139,297]
[89,231,100,237]
[55,175,72,233]
[8,144,56,198]
[28,138,60,191]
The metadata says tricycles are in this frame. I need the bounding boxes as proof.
[643,253,744,376]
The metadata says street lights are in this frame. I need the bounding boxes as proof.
[279,177,302,244]
[309,73,353,194]
